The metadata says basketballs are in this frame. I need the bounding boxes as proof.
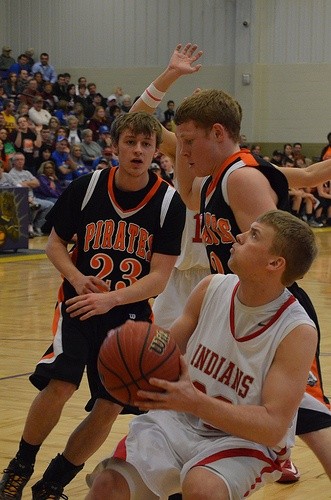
[99,321,181,405]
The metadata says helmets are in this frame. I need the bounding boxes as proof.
[99,126,111,134]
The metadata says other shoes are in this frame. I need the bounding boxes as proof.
[34,225,41,234]
[307,220,323,228]
[28,223,33,233]
[322,220,326,227]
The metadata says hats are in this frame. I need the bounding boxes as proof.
[56,135,68,142]
[98,158,109,165]
[273,149,282,156]
[31,96,46,103]
[108,95,117,102]
[2,46,12,51]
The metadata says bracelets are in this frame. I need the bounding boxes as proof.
[140,82,167,109]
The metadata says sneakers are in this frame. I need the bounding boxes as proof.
[32,478,68,500]
[275,458,300,482]
[0,457,34,500]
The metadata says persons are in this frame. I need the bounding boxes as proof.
[86,209,318,500]
[127,43,331,485]
[0,113,187,500]
[0,46,331,238]
